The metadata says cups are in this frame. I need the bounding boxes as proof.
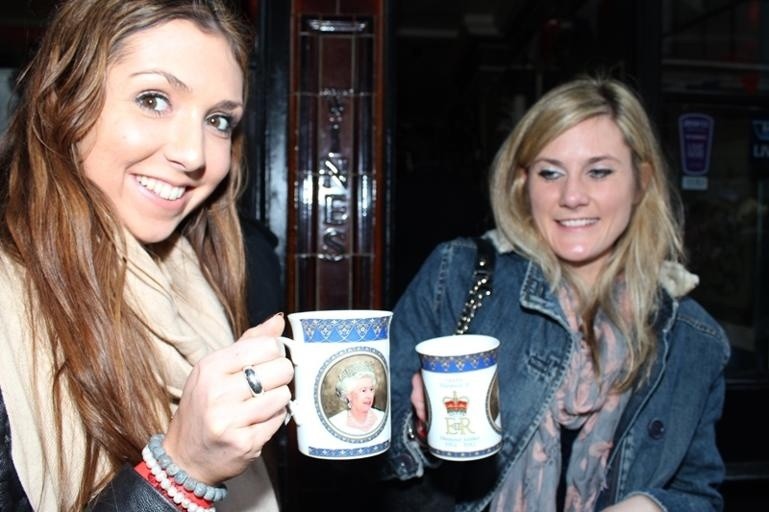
[274,309,397,462]
[415,335,505,462]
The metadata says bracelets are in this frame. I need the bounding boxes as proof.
[142,443,217,512]
[148,432,229,502]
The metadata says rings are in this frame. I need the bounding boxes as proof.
[241,363,265,398]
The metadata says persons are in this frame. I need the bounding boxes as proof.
[328,359,386,436]
[0,1,300,512]
[384,72,735,511]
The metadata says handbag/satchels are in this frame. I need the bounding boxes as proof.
[297,239,496,512]
